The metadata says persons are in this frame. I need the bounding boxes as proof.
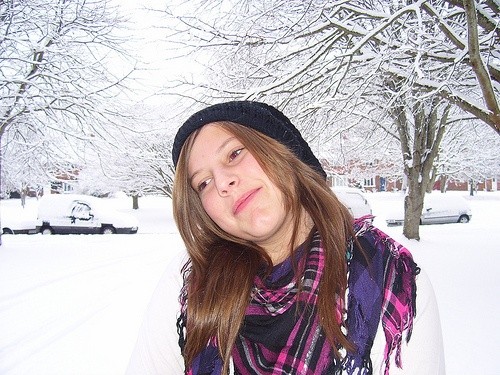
[126,100,445,375]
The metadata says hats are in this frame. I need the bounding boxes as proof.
[172,101,328,183]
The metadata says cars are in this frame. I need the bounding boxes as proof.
[385,197,472,225]
[1,194,139,234]
